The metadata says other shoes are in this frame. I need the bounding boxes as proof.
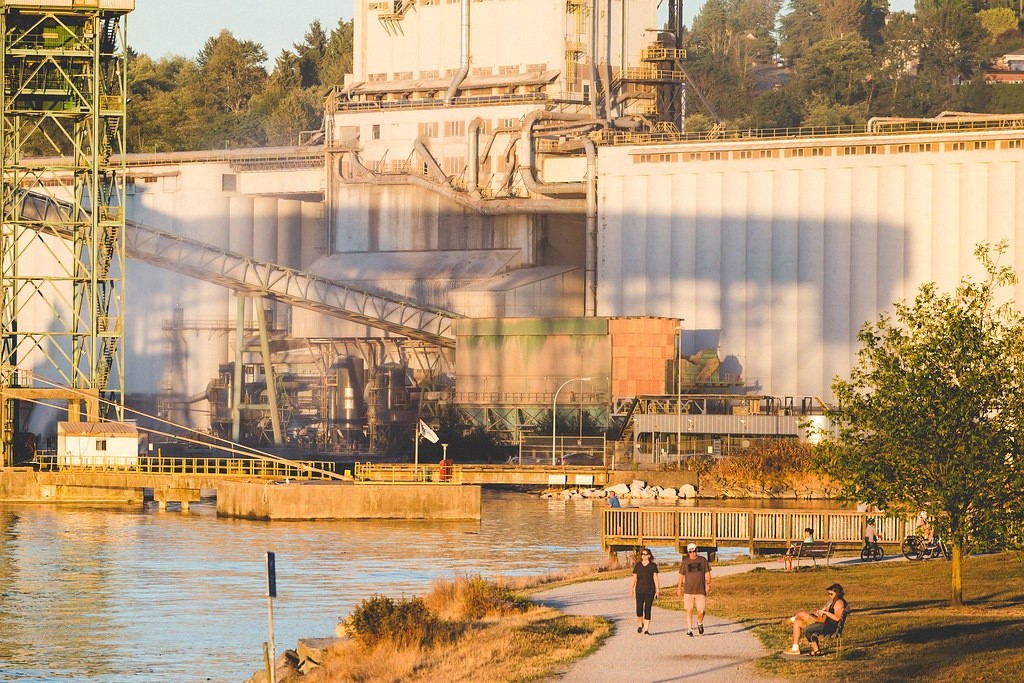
[784,618,794,624]
[686,632,692,636]
[782,648,801,655]
[645,631,649,635]
[638,623,644,633]
[697,622,703,634]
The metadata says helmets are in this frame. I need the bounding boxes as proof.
[867,518,874,525]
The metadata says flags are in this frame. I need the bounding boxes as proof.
[419,420,439,444]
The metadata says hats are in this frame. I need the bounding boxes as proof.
[687,543,697,551]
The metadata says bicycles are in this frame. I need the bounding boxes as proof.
[901,520,951,561]
[860,536,884,562]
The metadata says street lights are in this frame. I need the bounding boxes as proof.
[675,327,685,471]
[553,377,592,465]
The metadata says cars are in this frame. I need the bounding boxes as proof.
[556,453,603,466]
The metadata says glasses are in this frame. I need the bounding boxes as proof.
[641,553,649,556]
[689,550,696,553]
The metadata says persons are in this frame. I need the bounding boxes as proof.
[857,499,936,559]
[778,528,814,571]
[678,543,712,636]
[608,491,623,536]
[782,583,847,656]
[631,549,660,635]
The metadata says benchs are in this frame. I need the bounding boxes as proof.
[809,605,851,659]
[782,540,838,572]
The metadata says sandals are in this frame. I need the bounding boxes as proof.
[806,649,821,656]
